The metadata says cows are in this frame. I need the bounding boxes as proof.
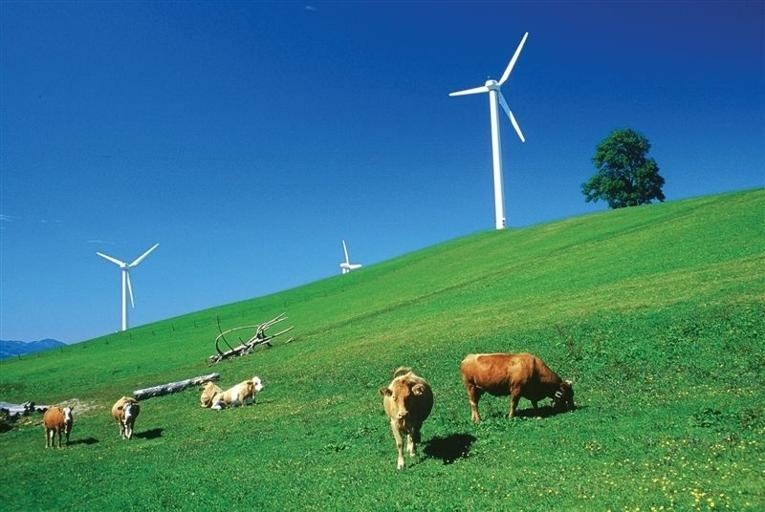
[378,366,434,471]
[112,396,140,440]
[460,352,575,424]
[43,406,74,448]
[200,376,264,411]
